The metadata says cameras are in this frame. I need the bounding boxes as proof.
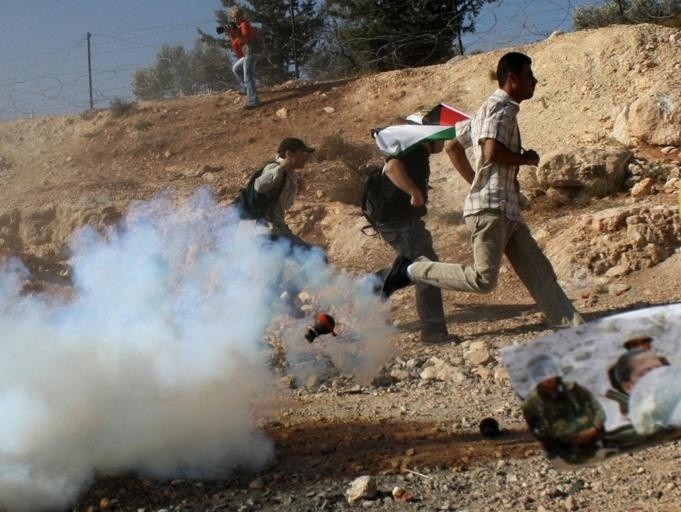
[217,22,236,35]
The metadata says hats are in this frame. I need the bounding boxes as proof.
[278,137,316,155]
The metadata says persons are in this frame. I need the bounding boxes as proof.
[381,52,586,332]
[371,139,459,346]
[231,138,328,318]
[522,375,606,464]
[224,5,259,109]
[606,335,671,398]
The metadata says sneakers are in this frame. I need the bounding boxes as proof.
[380,254,415,304]
[239,92,260,110]
[422,333,460,345]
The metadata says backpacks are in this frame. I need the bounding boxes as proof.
[360,146,430,230]
[234,161,288,220]
